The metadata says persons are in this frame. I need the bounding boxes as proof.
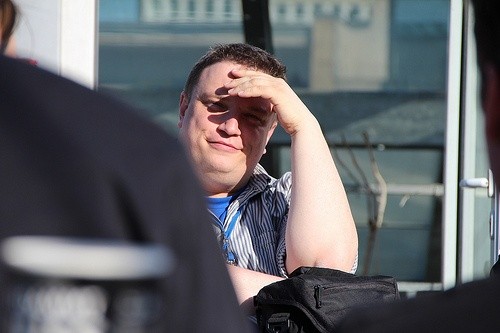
[0,1,249,333]
[334,1,500,333]
[176,42,360,307]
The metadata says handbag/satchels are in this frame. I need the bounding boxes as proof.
[252,266,401,333]
